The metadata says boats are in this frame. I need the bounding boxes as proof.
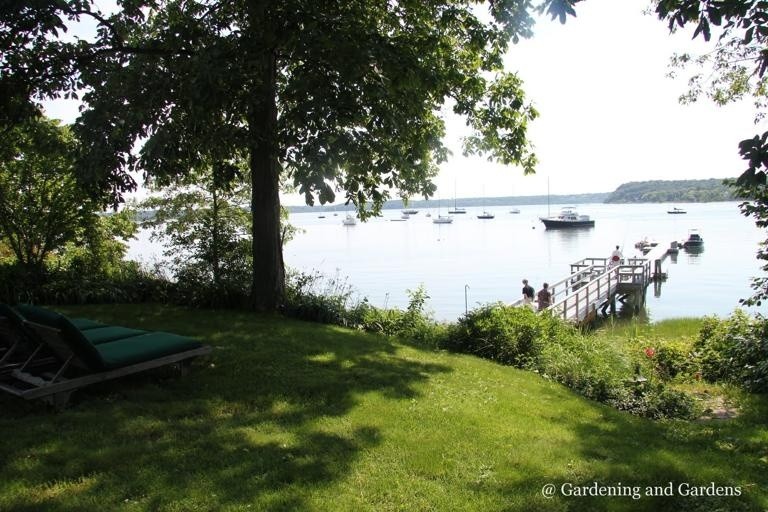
[425,212,431,217]
[667,207,687,215]
[539,176,597,229]
[476,210,496,220]
[318,216,326,219]
[343,214,357,225]
[508,208,521,214]
[333,213,338,217]
[682,228,704,247]
[389,208,420,222]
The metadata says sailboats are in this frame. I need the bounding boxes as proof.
[432,183,467,224]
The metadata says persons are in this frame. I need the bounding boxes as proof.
[536,282,553,312]
[610,244,624,278]
[521,278,535,304]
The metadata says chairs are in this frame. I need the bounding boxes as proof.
[2,293,211,400]
